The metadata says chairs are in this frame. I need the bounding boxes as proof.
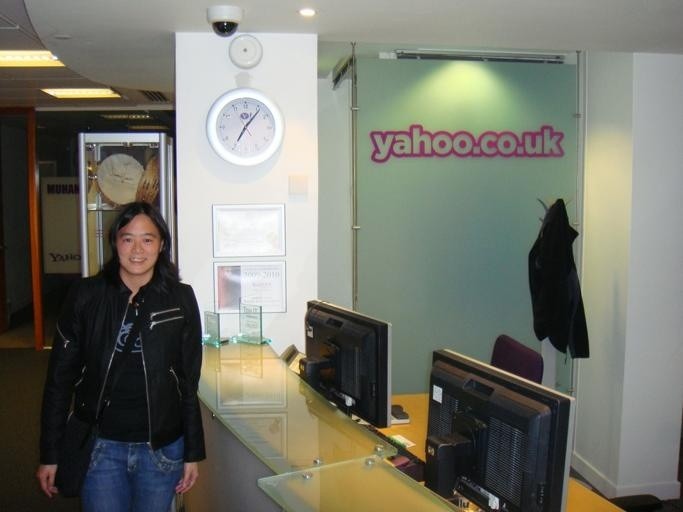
[489,334,544,385]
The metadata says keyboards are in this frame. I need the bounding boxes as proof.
[361,424,424,481]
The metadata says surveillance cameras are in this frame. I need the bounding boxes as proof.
[208,7,243,37]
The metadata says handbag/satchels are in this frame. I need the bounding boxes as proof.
[54,412,98,497]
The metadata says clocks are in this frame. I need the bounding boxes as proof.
[207,87,285,167]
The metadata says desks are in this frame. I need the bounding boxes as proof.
[373,394,627,512]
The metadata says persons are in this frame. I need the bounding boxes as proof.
[35,200,206,510]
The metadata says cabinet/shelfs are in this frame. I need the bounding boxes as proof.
[79,131,174,277]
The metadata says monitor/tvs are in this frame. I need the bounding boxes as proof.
[298,299,391,428]
[424,349,575,512]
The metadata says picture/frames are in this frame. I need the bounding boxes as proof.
[213,261,287,313]
[212,204,286,257]
